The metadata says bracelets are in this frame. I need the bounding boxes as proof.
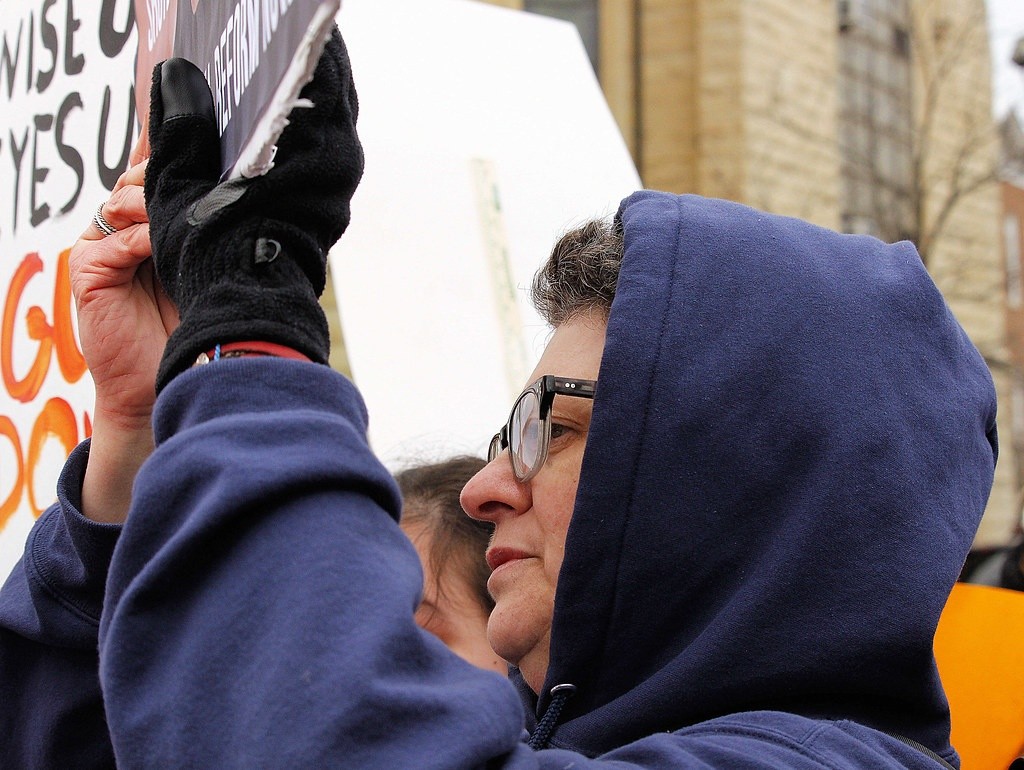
[189,340,315,382]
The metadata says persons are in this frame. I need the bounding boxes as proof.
[389,456,514,689]
[0,19,999,770]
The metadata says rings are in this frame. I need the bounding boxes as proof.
[93,201,119,238]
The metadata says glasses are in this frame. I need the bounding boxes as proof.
[485,373,599,484]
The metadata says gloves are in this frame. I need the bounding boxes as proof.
[145,19,360,384]
